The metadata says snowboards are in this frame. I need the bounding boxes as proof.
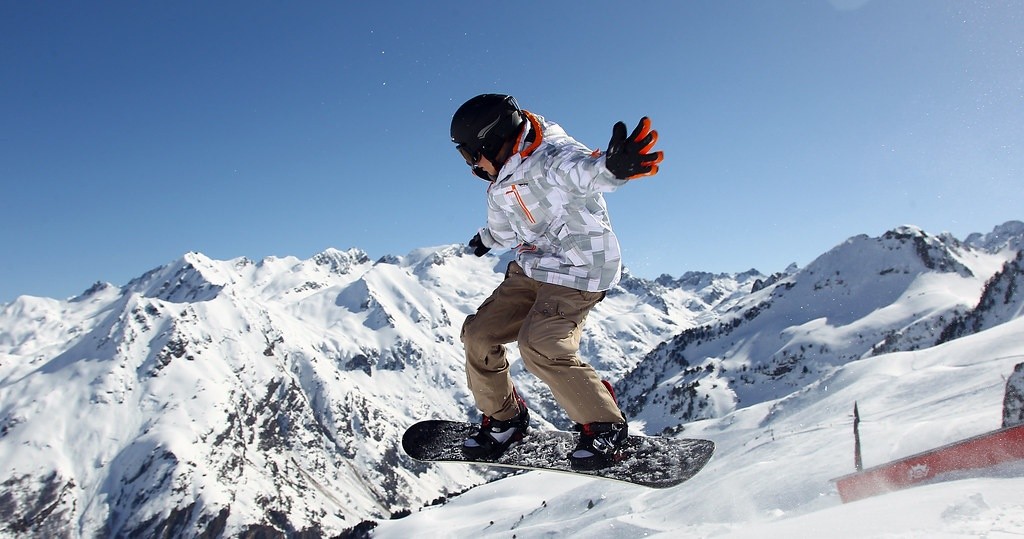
[402,419,716,490]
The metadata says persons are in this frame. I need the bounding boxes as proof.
[450,94,663,464]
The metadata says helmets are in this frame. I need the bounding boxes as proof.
[450,93,527,182]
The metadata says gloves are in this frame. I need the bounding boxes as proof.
[468,232,491,257]
[606,116,664,180]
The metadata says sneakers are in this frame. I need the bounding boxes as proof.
[571,412,629,461]
[462,398,531,457]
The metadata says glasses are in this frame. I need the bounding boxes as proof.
[455,144,479,166]
